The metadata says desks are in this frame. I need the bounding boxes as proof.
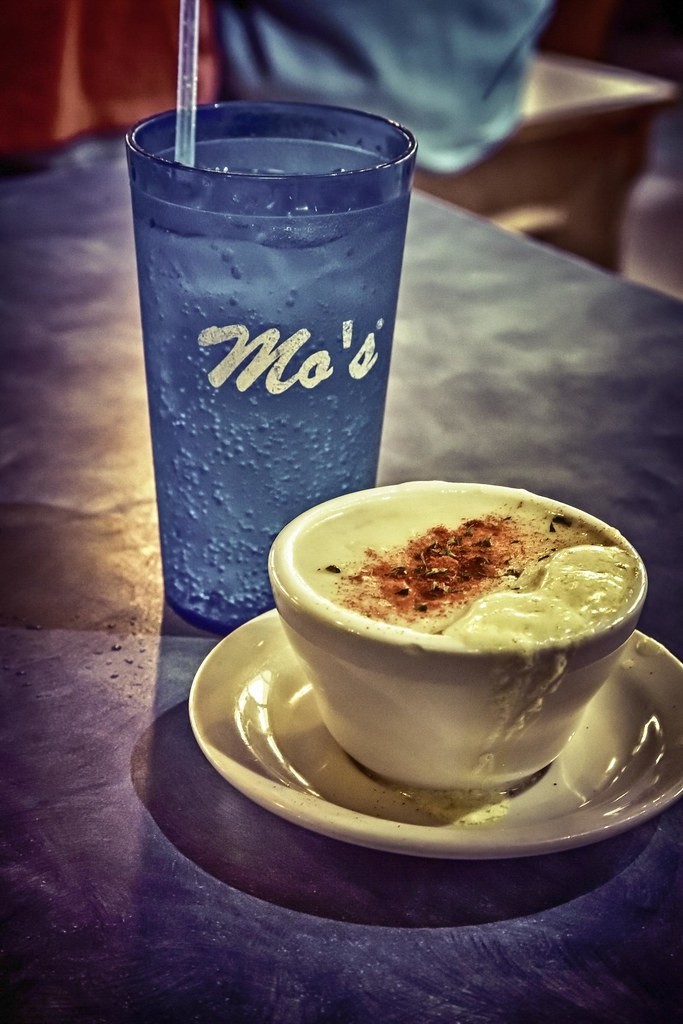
[0,119,683,1024]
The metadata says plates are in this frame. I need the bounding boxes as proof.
[187,606,683,862]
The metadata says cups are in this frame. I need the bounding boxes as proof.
[267,479,649,790]
[123,99,418,635]
